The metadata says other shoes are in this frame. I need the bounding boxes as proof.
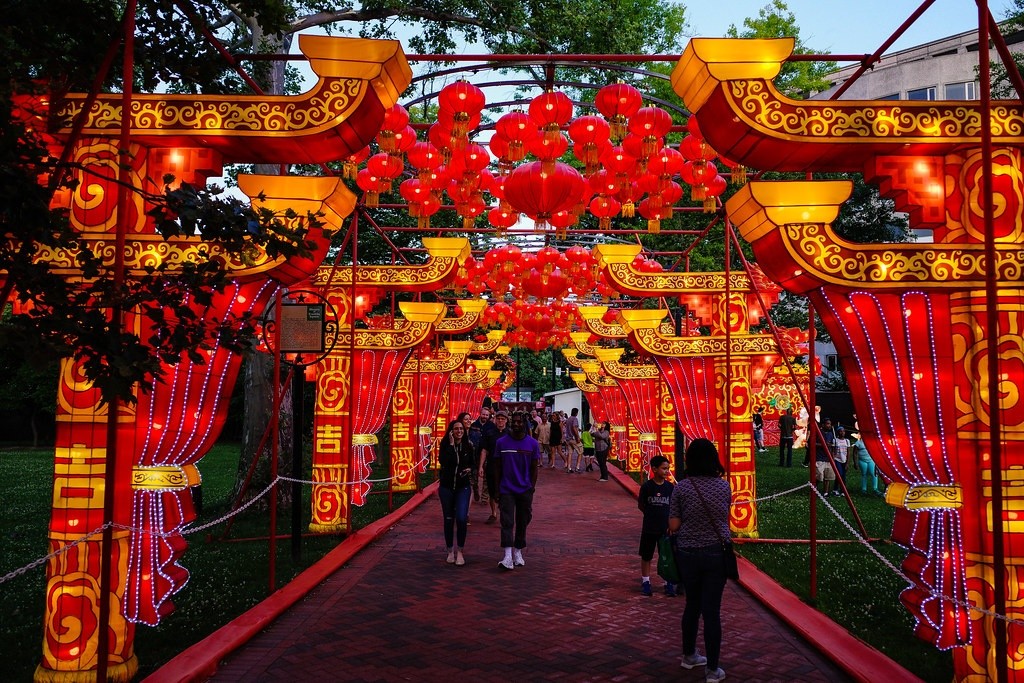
[586,466,589,471]
[446,552,455,563]
[485,515,497,523]
[467,516,471,526]
[564,463,568,468]
[588,464,593,471]
[474,490,480,501]
[567,469,574,473]
[455,551,465,565]
[537,464,543,467]
[550,465,555,468]
[575,468,582,472]
[599,478,608,481]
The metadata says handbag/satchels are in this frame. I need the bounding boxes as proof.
[720,549,739,581]
[657,537,679,585]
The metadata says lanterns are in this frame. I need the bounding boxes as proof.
[449,244,664,356]
[341,78,748,242]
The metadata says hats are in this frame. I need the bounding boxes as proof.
[496,411,508,418]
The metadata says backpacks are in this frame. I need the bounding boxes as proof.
[604,438,612,447]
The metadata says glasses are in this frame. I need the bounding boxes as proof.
[452,427,464,430]
[497,417,506,420]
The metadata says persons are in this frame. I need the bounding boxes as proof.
[493,411,541,571]
[438,421,476,565]
[566,407,583,474]
[753,407,770,452]
[638,455,678,597]
[590,420,610,482]
[778,408,796,467]
[579,422,595,472]
[853,436,882,495]
[801,416,850,497]
[459,406,569,526]
[668,438,732,683]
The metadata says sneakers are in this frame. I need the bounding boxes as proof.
[664,583,674,596]
[680,648,707,670]
[497,554,514,569]
[705,666,726,683]
[639,581,652,595]
[514,552,525,566]
